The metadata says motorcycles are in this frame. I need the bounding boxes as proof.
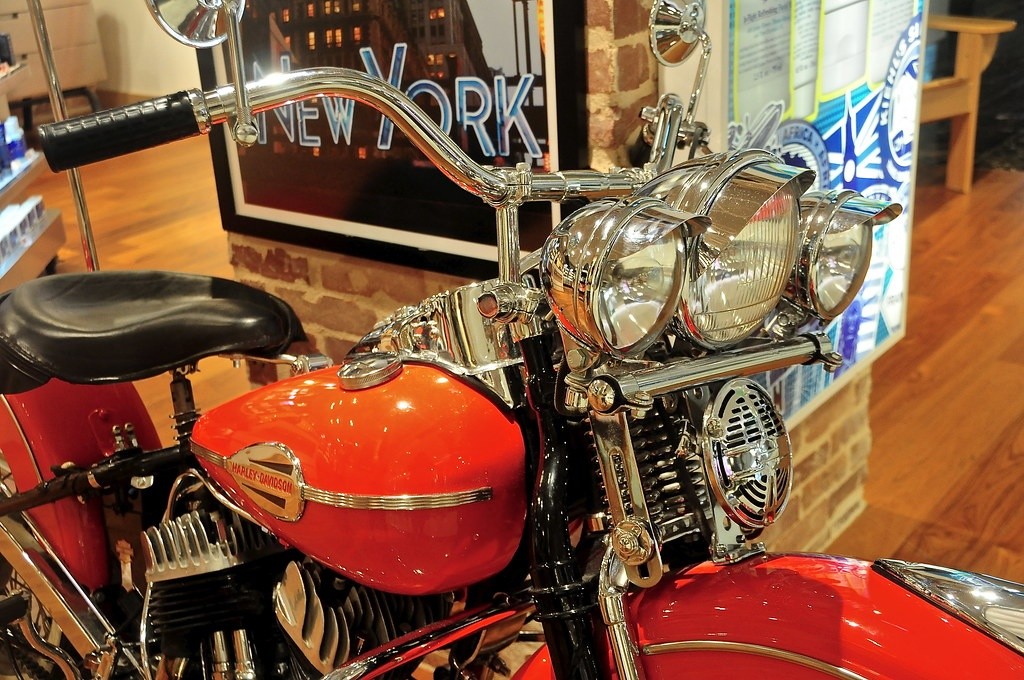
[0,0,1024,680]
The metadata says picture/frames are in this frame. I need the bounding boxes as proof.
[190,1,587,281]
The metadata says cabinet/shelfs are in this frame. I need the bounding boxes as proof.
[0,1,106,128]
